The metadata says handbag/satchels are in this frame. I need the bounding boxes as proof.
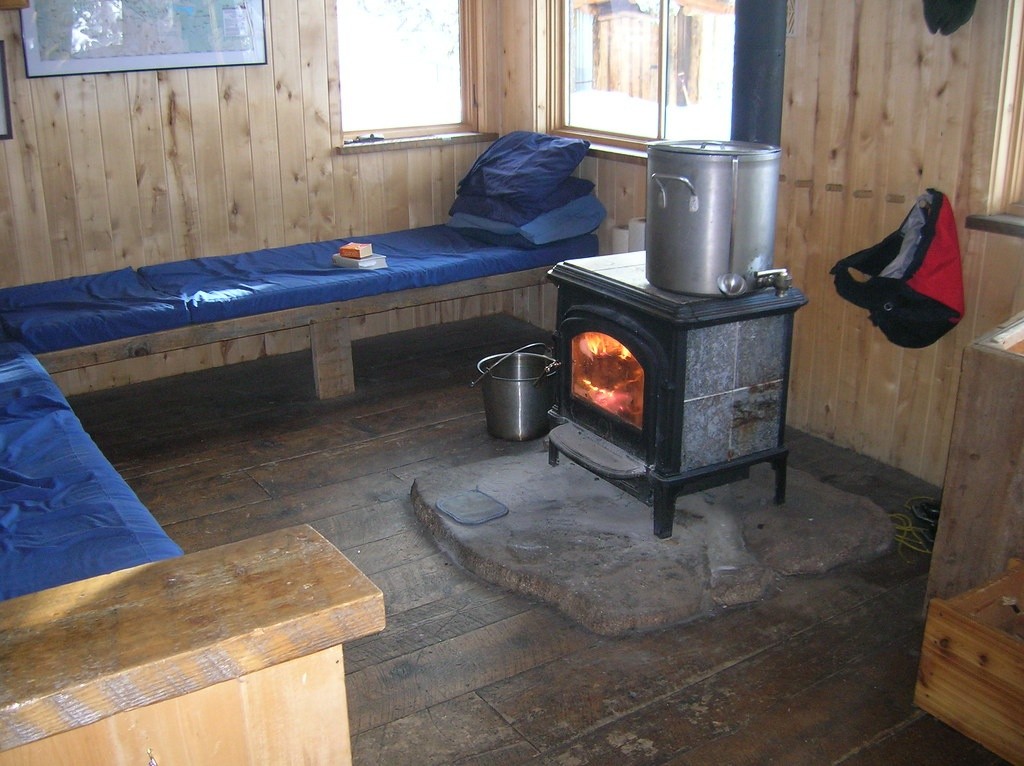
[830,186,963,347]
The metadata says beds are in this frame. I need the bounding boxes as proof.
[0,127,607,400]
[0,337,184,601]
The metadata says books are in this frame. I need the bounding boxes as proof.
[332,252,389,269]
[339,241,373,259]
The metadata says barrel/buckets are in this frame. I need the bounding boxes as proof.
[645,139,793,298]
[470,342,562,443]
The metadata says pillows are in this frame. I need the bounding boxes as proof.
[446,131,606,250]
